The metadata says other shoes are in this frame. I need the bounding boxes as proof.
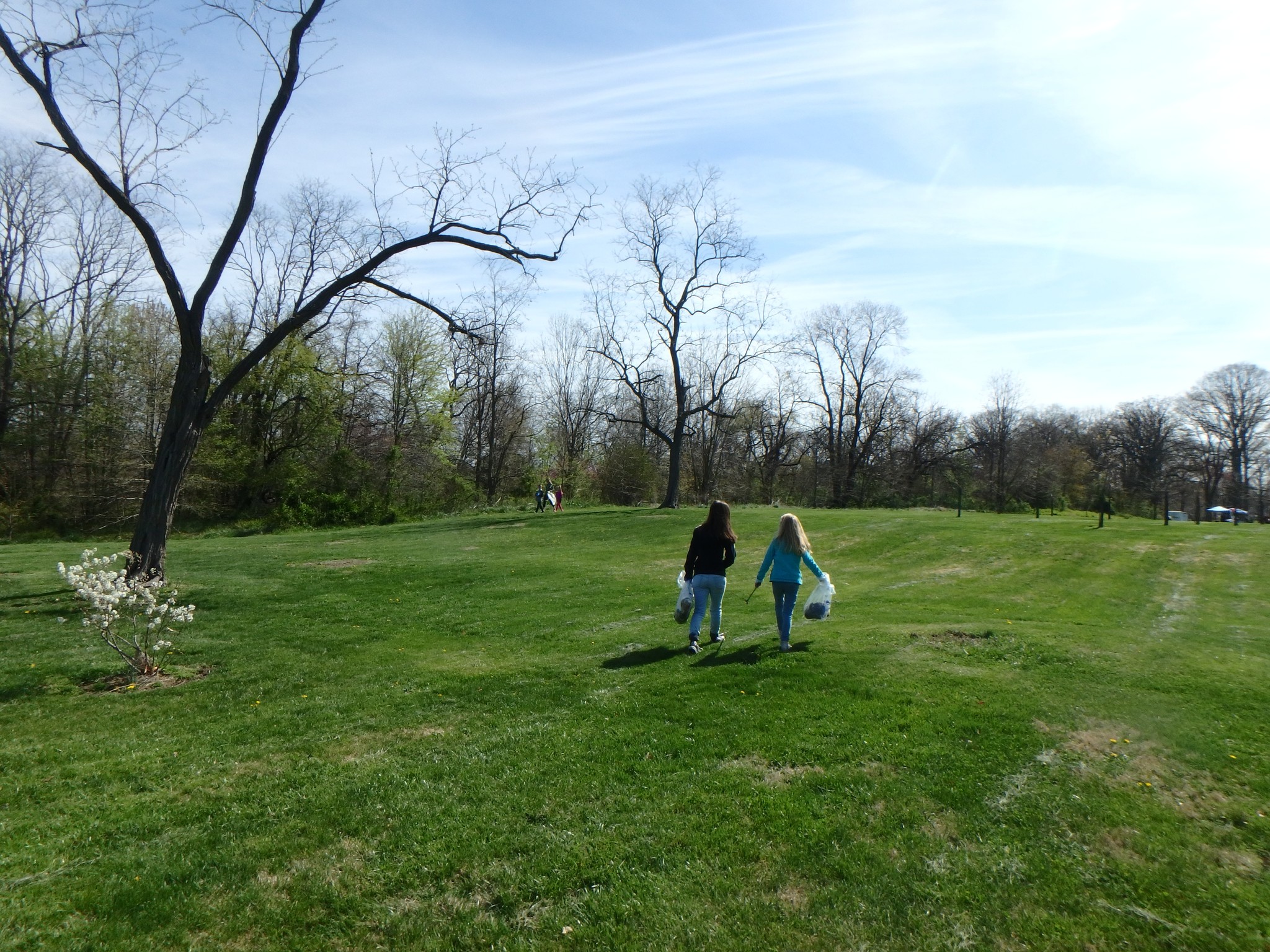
[554,510,556,512]
[780,641,791,650]
[540,509,545,512]
[553,508,556,511]
[688,642,703,654]
[541,511,544,513]
[561,510,564,511]
[710,634,725,642]
[534,511,536,513]
[777,629,782,638]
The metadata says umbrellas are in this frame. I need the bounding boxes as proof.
[1228,508,1250,513]
[1206,506,1231,512]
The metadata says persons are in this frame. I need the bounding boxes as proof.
[533,484,544,514]
[541,478,556,512]
[684,500,739,655]
[553,485,564,512]
[755,513,826,652]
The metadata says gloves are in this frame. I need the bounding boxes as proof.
[685,579,692,585]
[755,582,761,588]
